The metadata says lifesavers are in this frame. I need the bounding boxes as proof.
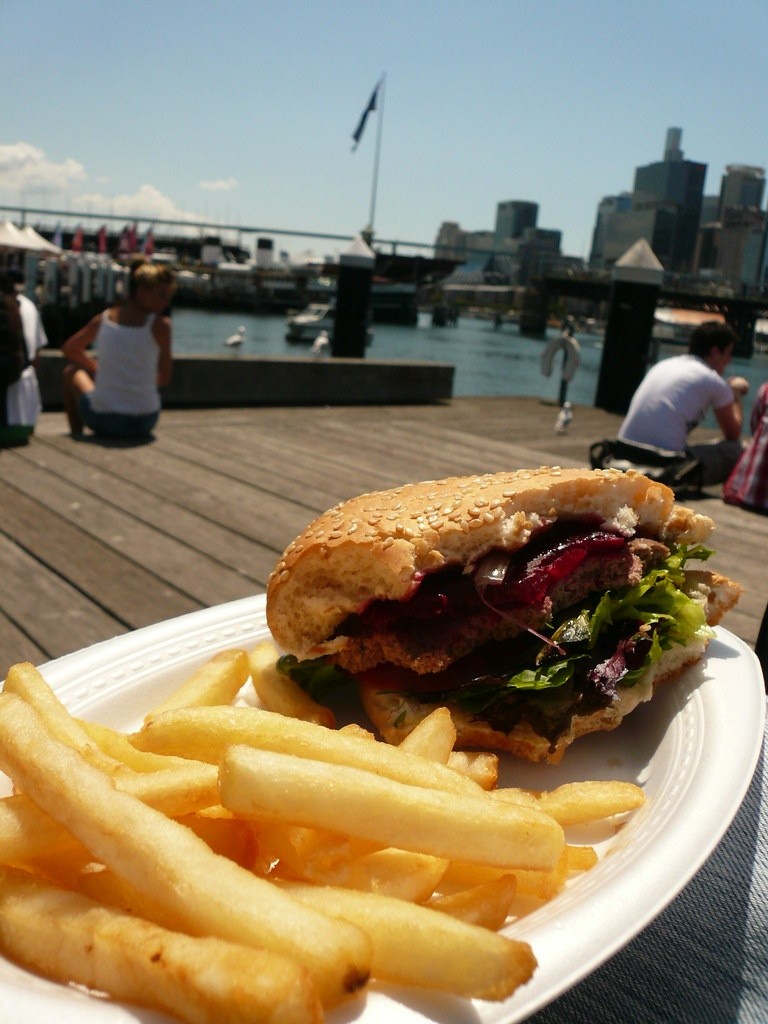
[539,335,584,381]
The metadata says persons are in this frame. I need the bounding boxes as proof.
[0,269,46,448]
[615,321,752,489]
[721,382,768,516]
[62,263,176,438]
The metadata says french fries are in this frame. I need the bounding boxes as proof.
[0,643,647,1024]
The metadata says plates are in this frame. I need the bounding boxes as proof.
[0,590,768,1024]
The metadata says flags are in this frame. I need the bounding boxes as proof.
[353,89,378,141]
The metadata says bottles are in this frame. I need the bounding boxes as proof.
[555,401,574,433]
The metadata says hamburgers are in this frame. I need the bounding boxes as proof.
[265,467,743,763]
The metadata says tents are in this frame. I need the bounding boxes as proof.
[0,218,62,256]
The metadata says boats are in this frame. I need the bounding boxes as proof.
[286,302,375,346]
